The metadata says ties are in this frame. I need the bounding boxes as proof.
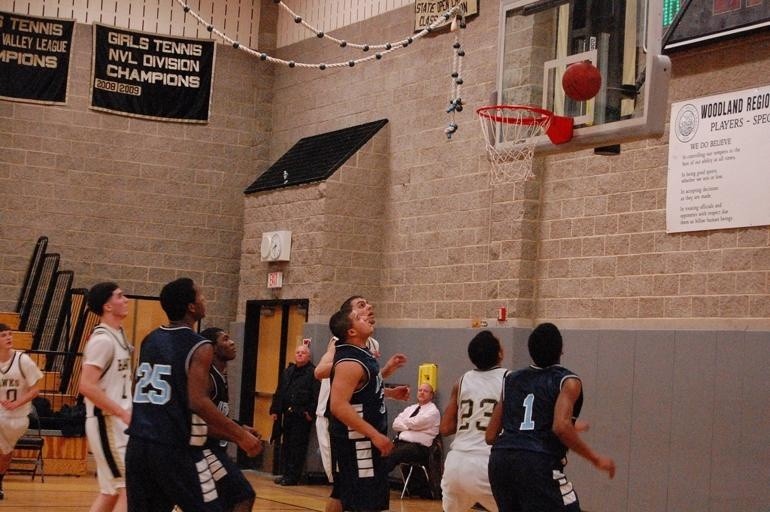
[409,406,421,418]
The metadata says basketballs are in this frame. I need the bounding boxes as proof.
[562,62,601,100]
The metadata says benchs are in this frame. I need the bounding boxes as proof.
[0,311,90,475]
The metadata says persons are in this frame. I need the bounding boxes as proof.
[439,323,616,512]
[0,323,45,499]
[314,296,410,512]
[390,383,441,475]
[269,345,321,485]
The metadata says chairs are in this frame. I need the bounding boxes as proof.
[1,405,46,482]
[397,433,443,500]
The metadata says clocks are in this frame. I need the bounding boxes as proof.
[269,234,282,260]
